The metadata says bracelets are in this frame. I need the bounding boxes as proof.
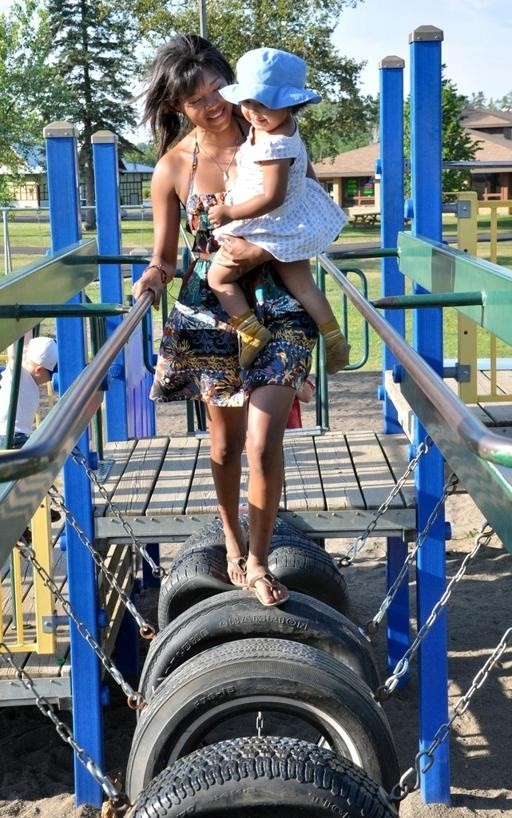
[142,264,167,284]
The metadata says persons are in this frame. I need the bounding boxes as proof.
[0,334,61,548]
[207,47,351,374]
[128,30,338,608]
[284,379,314,429]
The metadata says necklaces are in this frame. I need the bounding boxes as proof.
[193,116,242,182]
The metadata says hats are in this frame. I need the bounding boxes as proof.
[217,44,323,114]
[26,335,65,375]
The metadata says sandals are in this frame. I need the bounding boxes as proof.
[247,564,290,608]
[225,540,255,589]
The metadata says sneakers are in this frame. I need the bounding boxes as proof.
[229,311,270,366]
[320,319,352,373]
